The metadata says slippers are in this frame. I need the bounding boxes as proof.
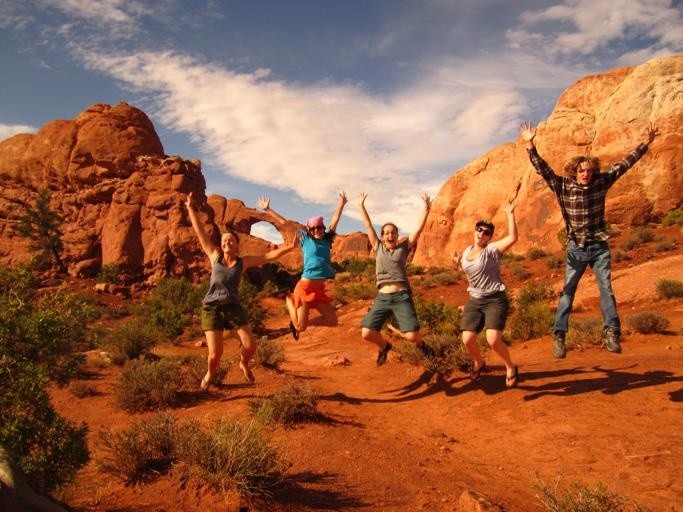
[240,367,254,382]
[201,372,215,391]
[470,361,485,380]
[506,366,518,387]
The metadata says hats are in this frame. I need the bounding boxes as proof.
[475,220,494,234]
[307,216,323,227]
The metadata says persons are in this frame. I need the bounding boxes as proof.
[448,198,520,390]
[516,119,659,360]
[182,192,301,394]
[254,192,347,339]
[357,189,436,367]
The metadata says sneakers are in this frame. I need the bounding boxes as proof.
[552,332,565,358]
[417,342,435,359]
[605,328,621,353]
[268,287,291,299]
[290,319,299,339]
[377,343,392,364]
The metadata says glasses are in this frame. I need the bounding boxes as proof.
[310,226,322,231]
[477,227,491,236]
